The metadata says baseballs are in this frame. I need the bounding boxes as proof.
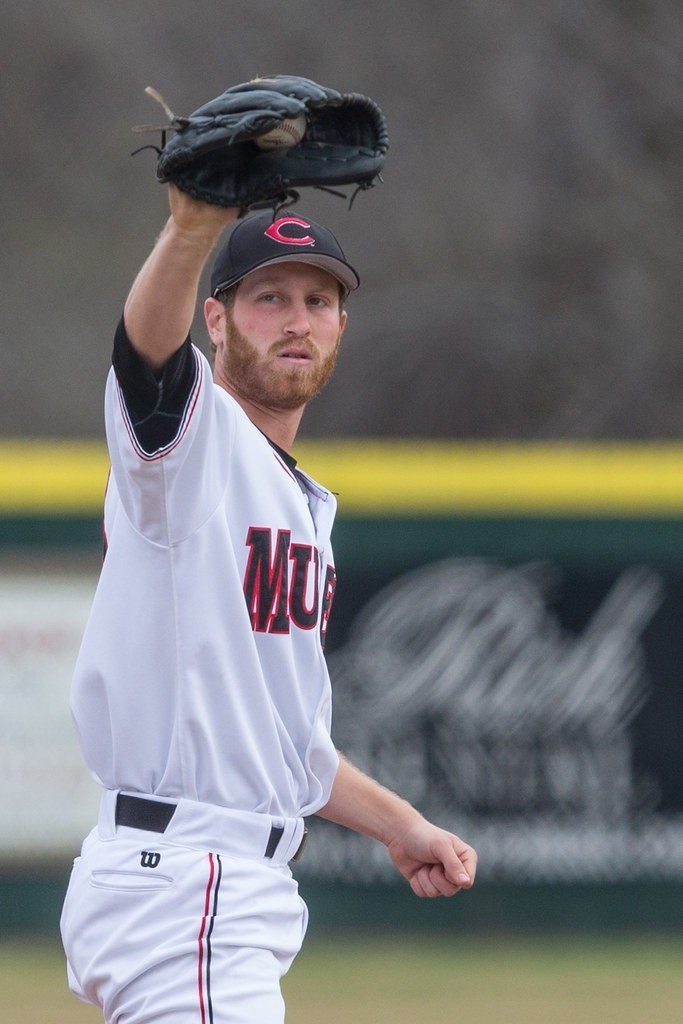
[255,112,306,150]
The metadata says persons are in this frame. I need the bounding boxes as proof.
[60,75,479,1024]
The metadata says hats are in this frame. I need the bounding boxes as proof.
[211,209,360,307]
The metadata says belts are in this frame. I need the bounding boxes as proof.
[115,793,309,862]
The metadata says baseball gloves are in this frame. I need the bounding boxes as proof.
[156,77,390,206]
